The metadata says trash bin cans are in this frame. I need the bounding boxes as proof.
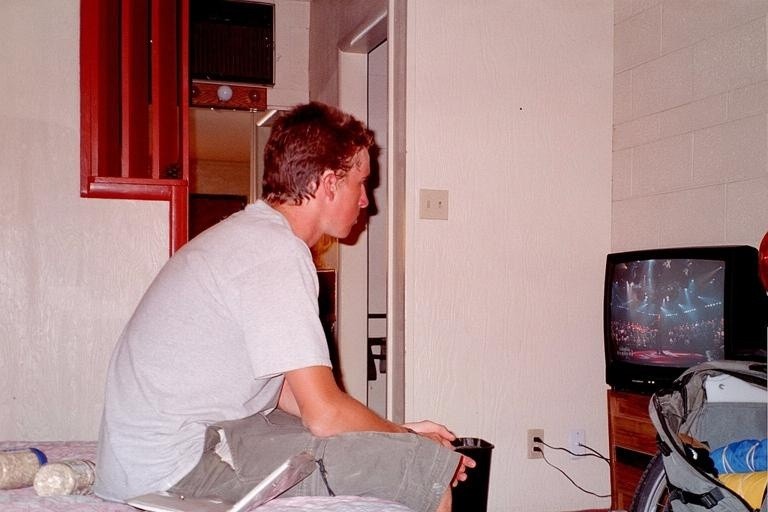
[450,438,494,512]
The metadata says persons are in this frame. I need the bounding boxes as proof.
[91,96,477,511]
[611,313,725,354]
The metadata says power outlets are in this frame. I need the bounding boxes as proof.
[525,427,545,461]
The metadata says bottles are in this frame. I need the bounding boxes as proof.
[33,461,95,497]
[0,449,47,489]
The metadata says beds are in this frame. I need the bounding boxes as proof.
[0,439,416,512]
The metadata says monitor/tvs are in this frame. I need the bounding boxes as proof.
[603,244,768,391]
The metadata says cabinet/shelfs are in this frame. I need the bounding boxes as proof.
[606,388,665,511]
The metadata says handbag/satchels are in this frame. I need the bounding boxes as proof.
[648,361,768,512]
